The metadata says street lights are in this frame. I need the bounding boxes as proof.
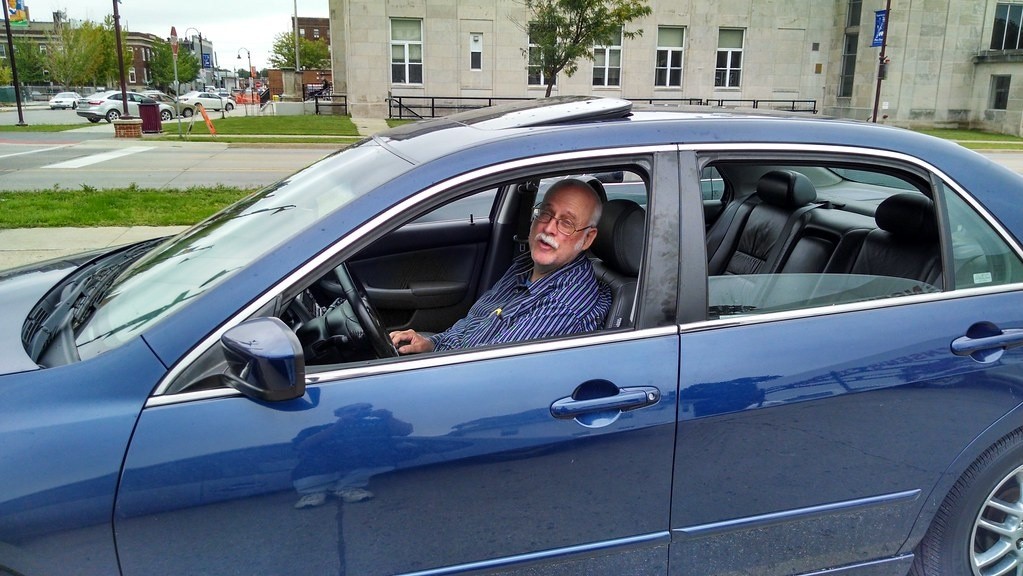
[183,27,205,92]
[237,48,255,105]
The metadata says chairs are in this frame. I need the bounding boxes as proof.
[586,198,647,330]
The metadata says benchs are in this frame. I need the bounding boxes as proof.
[706,169,987,314]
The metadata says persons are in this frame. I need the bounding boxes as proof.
[389,179,611,354]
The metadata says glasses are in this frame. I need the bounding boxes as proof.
[532,202,593,235]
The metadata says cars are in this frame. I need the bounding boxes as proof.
[76,90,176,123]
[0,96,1023,576]
[49,92,83,110]
[140,90,198,118]
[174,91,237,112]
[205,86,229,97]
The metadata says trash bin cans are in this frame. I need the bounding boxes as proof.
[137,98,163,134]
